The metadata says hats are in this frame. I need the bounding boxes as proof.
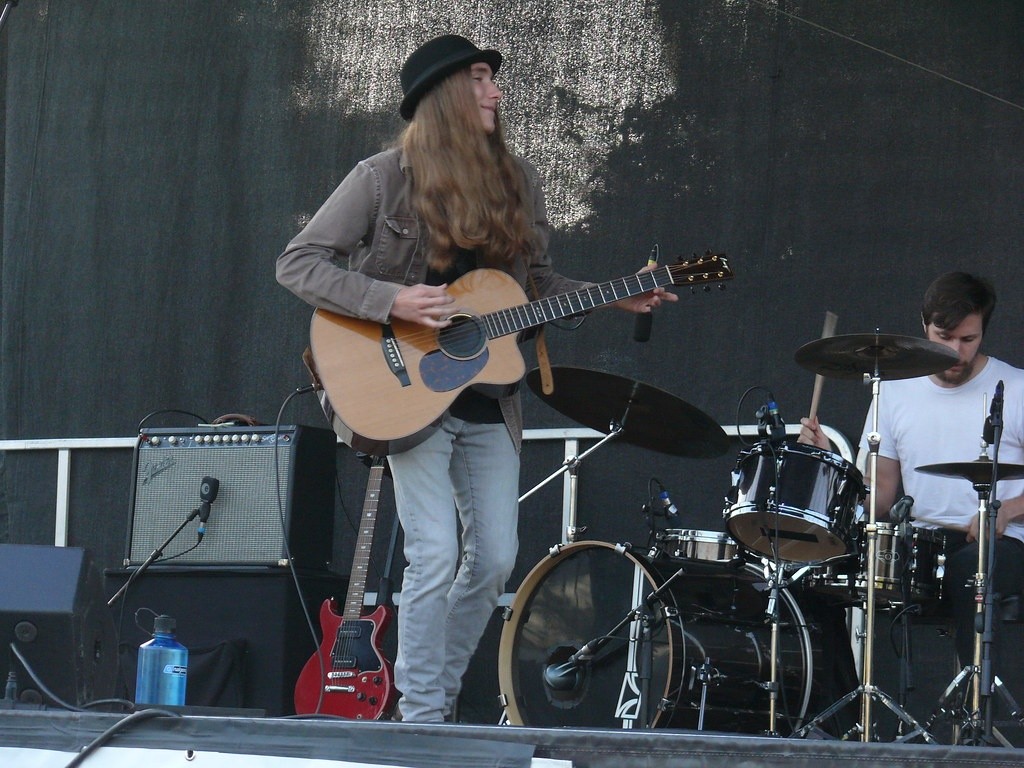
[401,35,504,121]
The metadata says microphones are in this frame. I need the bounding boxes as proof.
[197,476,219,543]
[632,247,659,343]
[543,664,584,690]
[889,495,914,521]
[659,485,681,528]
[766,392,786,439]
[983,380,1004,444]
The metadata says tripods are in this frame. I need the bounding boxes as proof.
[788,410,1024,753]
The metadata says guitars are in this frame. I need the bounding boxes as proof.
[308,250,738,455]
[294,457,395,718]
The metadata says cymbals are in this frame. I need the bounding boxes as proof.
[914,459,1022,484]
[797,334,961,382]
[524,362,731,458]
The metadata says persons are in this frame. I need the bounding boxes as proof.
[277,32,678,724]
[798,271,1024,727]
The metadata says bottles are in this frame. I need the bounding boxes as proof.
[135,607,188,704]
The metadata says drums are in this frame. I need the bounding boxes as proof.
[655,526,740,563]
[724,435,865,567]
[819,518,948,606]
[495,540,813,737]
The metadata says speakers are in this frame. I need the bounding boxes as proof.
[0,542,120,713]
[125,426,335,569]
